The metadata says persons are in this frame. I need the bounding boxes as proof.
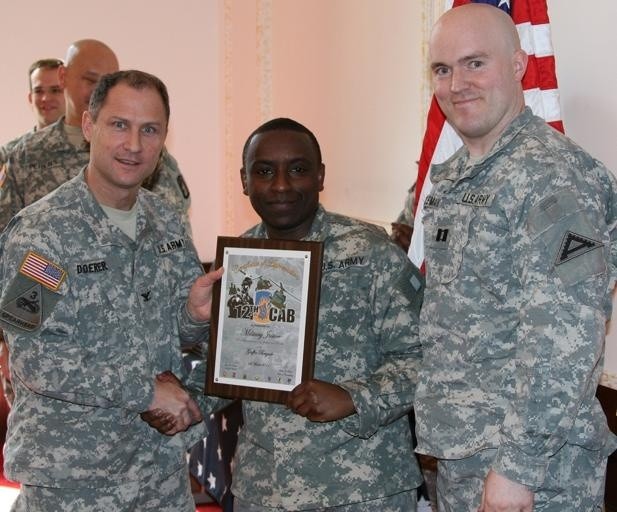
[0,59,65,169]
[0,69,228,512]
[411,3,617,511]
[0,38,192,416]
[139,117,427,511]
[387,182,419,253]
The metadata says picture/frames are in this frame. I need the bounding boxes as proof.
[204,236,324,404]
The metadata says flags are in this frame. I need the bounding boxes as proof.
[403,0,565,279]
